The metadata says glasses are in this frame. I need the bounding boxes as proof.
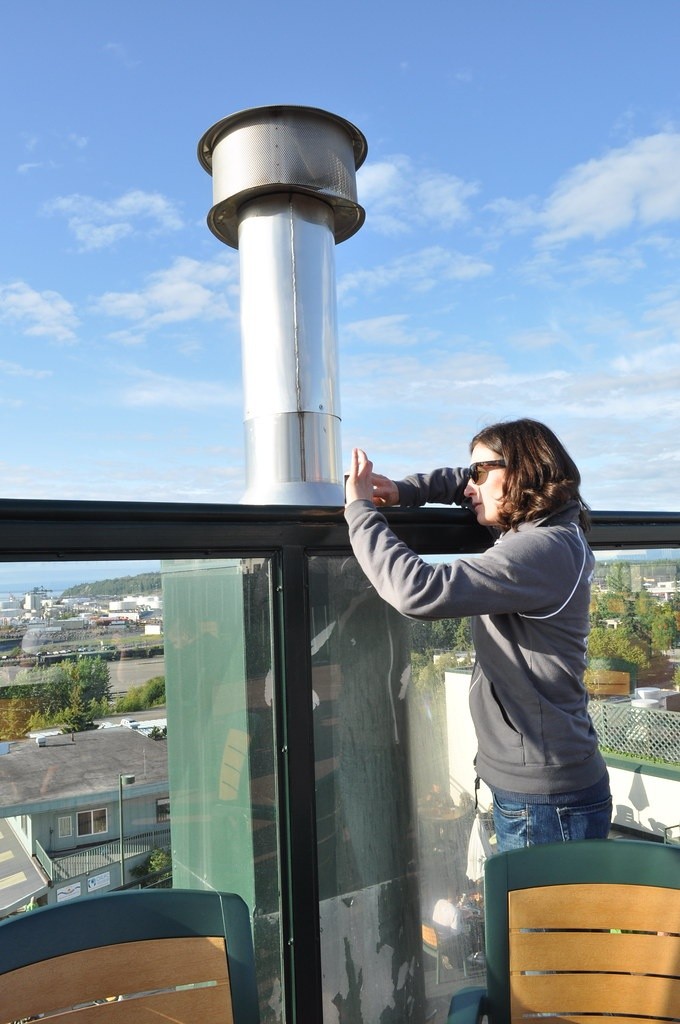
[464,459,505,485]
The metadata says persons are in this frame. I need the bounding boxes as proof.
[432,882,484,972]
[422,781,480,852]
[344,418,616,1018]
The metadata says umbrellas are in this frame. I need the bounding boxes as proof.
[466,814,493,913]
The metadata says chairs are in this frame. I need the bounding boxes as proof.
[0,889,253,1024]
[448,837,679,1023]
